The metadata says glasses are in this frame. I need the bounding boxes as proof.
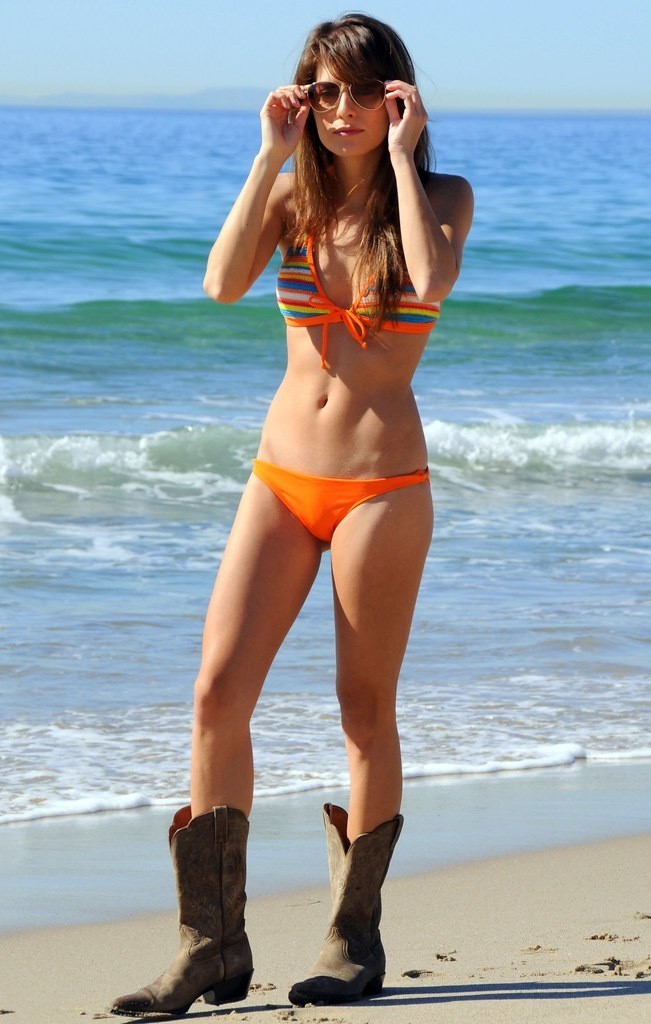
[305,77,388,113]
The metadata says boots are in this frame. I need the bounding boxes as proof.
[109,807,255,1019]
[288,802,402,1007]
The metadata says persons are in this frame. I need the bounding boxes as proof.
[112,13,475,1017]
